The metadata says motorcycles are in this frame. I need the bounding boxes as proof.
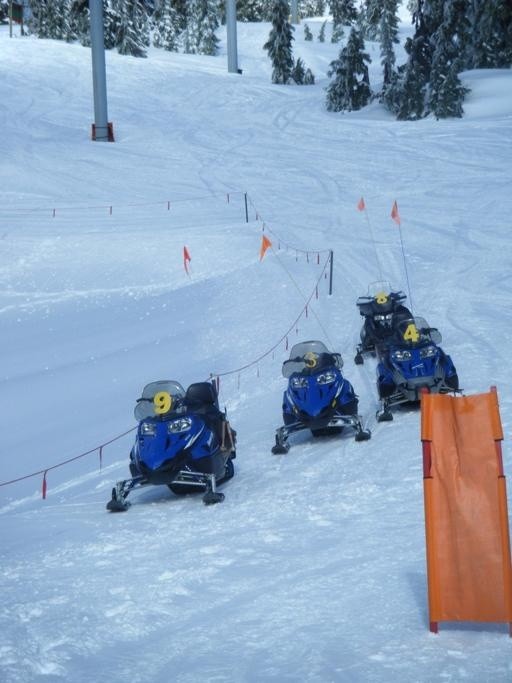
[107,379,238,514]
[272,340,371,454]
[354,280,460,421]
